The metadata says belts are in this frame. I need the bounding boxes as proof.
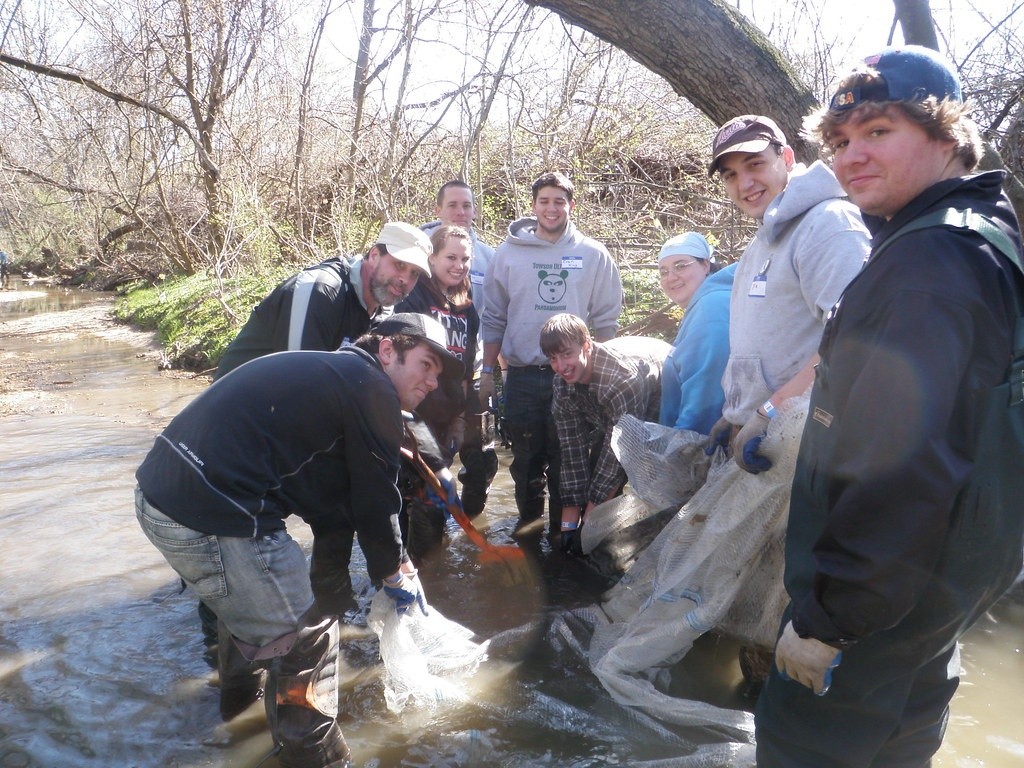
[511,365,552,372]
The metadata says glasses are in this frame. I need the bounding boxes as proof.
[659,258,698,278]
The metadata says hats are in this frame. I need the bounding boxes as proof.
[376,222,433,278]
[826,46,963,112]
[368,312,466,383]
[658,232,710,264]
[707,114,787,178]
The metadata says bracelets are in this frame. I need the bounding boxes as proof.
[561,522,576,528]
[483,368,493,371]
[385,573,402,583]
[763,400,776,417]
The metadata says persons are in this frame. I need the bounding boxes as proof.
[755,45,1024,768]
[396,226,480,562]
[709,115,869,691]
[471,172,622,523]
[0,249,10,281]
[657,233,737,437]
[133,312,465,768]
[212,222,432,592]
[538,313,674,553]
[420,180,507,507]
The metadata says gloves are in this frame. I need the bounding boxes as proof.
[571,522,594,559]
[445,417,466,456]
[775,619,843,697]
[479,373,499,415]
[734,409,771,475]
[383,569,430,618]
[425,467,464,520]
[705,417,731,461]
[560,529,577,561]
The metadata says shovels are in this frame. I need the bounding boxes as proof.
[400,419,528,588]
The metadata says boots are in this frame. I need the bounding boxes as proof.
[228,611,350,768]
[217,619,265,723]
